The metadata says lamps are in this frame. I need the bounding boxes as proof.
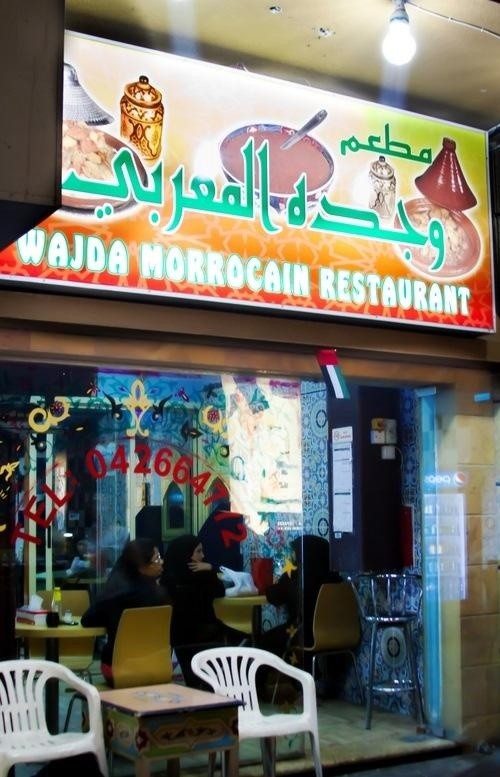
[381,0,500,68]
[63,531,75,538]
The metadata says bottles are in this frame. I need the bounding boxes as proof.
[120,75,164,168]
[51,586,62,624]
[368,156,396,222]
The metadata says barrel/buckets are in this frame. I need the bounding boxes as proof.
[250,557,274,594]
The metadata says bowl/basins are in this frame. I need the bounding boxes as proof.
[218,124,336,216]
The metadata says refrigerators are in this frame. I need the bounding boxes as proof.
[422,470,481,603]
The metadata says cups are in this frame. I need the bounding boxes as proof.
[65,607,72,625]
[46,612,59,627]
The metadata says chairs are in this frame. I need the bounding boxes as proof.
[0,660,109,775]
[347,571,425,731]
[25,590,95,686]
[62,604,172,734]
[271,583,362,709]
[191,646,323,776]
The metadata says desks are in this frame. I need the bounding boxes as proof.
[211,595,271,647]
[80,681,247,776]
[16,616,107,735]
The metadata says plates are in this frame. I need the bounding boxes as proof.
[392,197,481,278]
[60,128,148,214]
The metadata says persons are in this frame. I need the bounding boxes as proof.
[68,535,343,703]
